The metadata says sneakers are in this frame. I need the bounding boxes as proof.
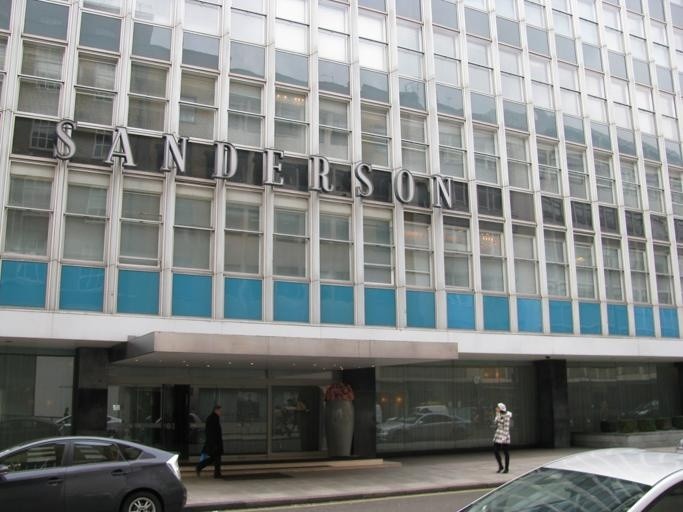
[496,466,504,473]
[195,464,201,477]
[502,467,509,473]
[214,474,226,479]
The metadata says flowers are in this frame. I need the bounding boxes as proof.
[319,380,355,403]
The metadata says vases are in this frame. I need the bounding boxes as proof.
[322,398,355,459]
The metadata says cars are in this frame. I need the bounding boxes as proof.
[454,445,682,512]
[631,398,659,418]
[374,412,473,442]
[0,412,122,450]
[0,432,191,510]
[150,412,207,435]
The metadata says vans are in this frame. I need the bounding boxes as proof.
[414,405,448,418]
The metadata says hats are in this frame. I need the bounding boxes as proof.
[498,402,506,413]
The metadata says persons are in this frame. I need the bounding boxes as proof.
[196,405,224,478]
[491,402,512,473]
